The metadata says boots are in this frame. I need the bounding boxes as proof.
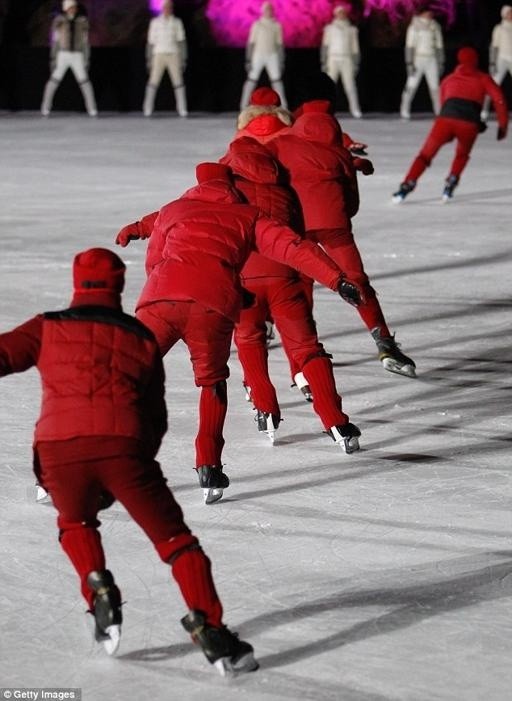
[181,609,254,664]
[370,326,416,368]
[392,179,416,200]
[443,176,458,199]
[86,569,123,642]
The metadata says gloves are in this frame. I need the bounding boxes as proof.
[116,221,143,247]
[338,277,368,307]
[349,142,368,156]
[354,158,373,175]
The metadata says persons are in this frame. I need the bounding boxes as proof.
[239,3,288,110]
[40,0,99,118]
[318,5,364,118]
[480,4,512,123]
[393,46,508,200]
[1,248,256,665]
[399,5,447,119]
[116,84,417,490]
[140,0,190,117]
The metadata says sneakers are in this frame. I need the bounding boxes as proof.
[293,372,312,396]
[198,465,229,488]
[257,405,279,431]
[327,423,361,441]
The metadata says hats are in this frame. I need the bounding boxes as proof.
[229,137,260,153]
[250,87,281,107]
[303,100,333,115]
[458,46,477,64]
[195,162,234,184]
[73,247,126,293]
